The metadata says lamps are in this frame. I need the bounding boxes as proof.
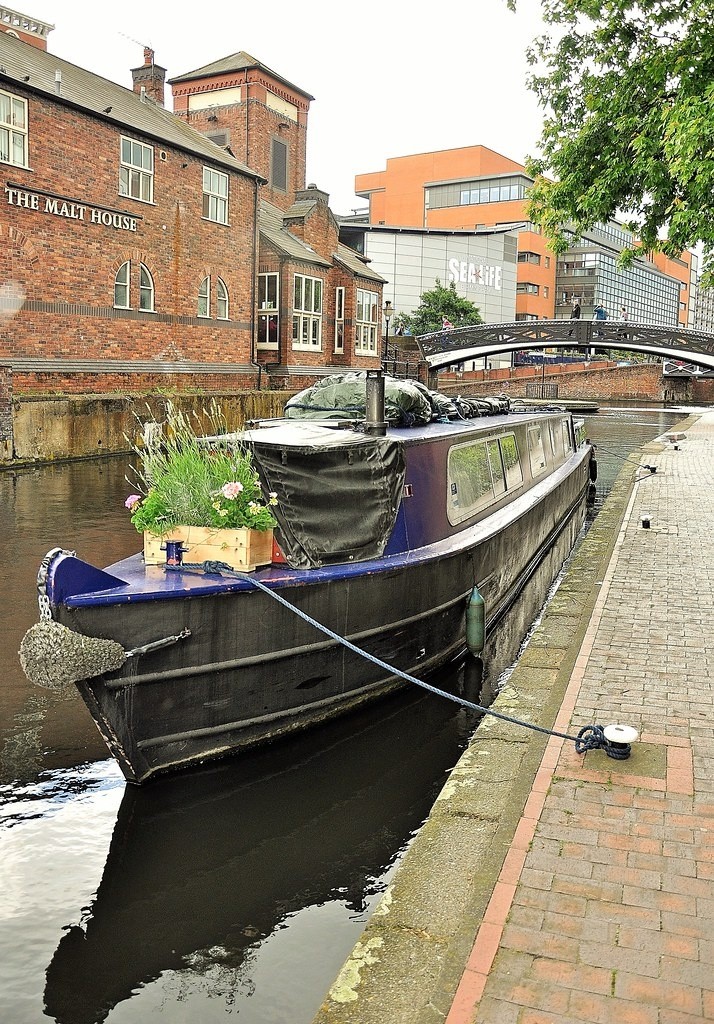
[278,123,289,129]
[207,114,218,122]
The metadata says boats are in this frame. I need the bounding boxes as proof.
[17,367,600,793]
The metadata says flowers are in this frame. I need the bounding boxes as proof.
[119,396,279,549]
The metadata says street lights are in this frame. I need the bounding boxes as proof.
[381,300,395,375]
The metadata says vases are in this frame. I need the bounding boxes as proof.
[141,525,275,573]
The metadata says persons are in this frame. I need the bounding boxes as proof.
[396,322,406,336]
[567,300,628,341]
[440,315,452,349]
